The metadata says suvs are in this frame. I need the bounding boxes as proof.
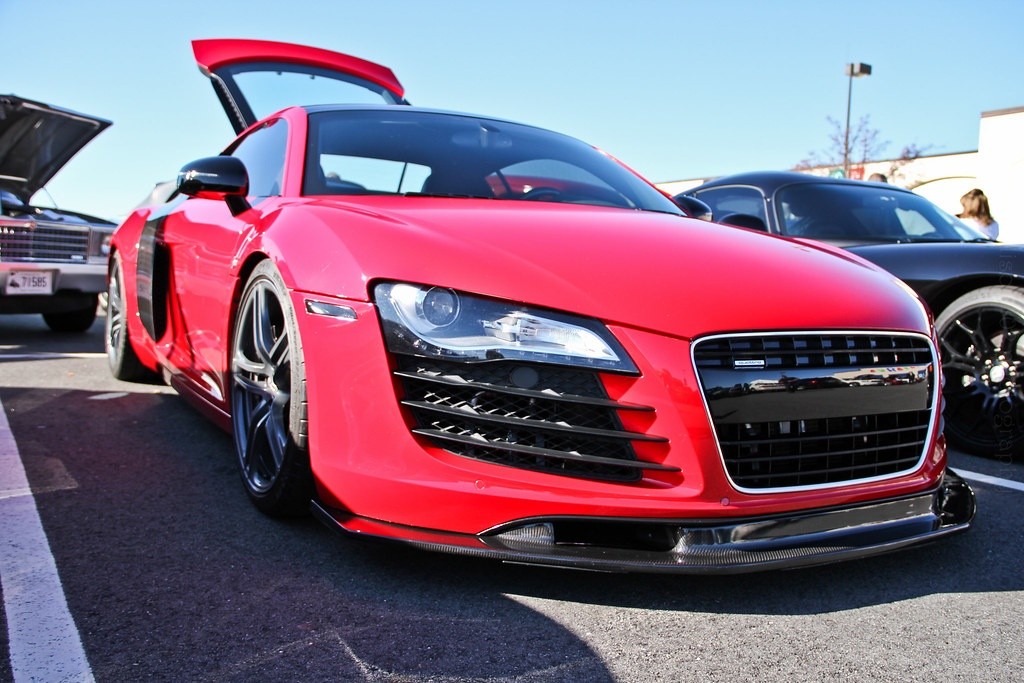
[0,94,122,337]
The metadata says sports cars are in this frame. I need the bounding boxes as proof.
[102,36,975,571]
[669,173,1024,456]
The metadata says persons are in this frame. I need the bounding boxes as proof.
[958,188,999,241]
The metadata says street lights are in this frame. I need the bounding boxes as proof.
[842,61,872,178]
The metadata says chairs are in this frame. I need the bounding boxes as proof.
[785,206,862,238]
[415,163,495,198]
[872,204,910,238]
